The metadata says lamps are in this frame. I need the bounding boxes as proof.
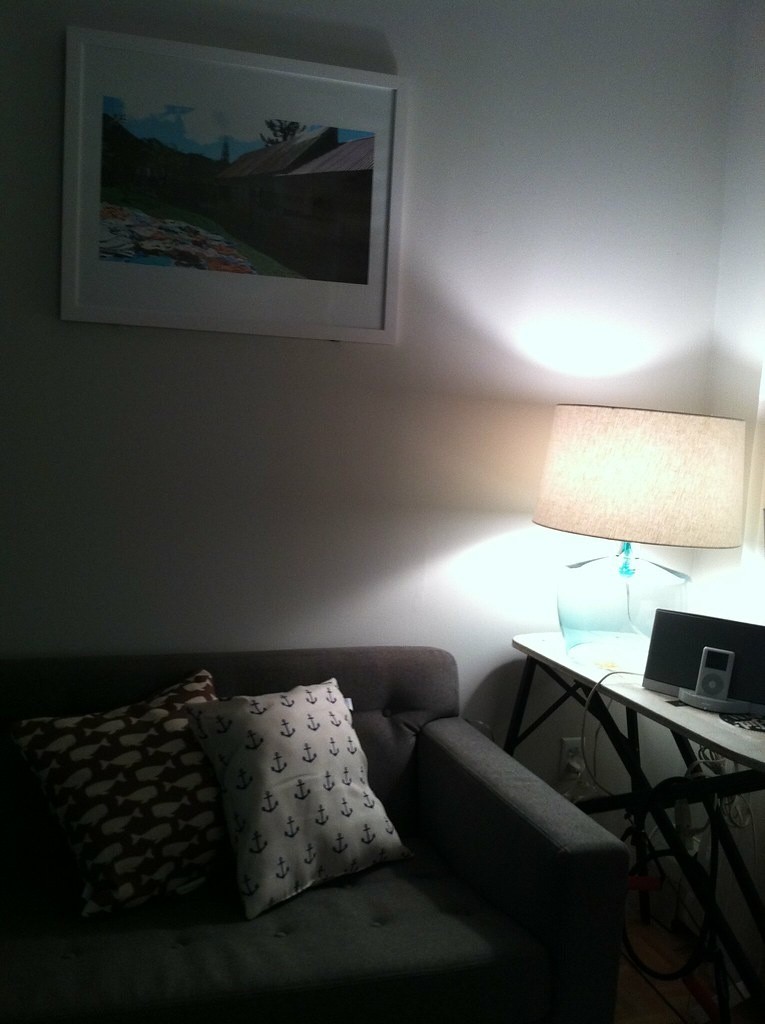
[525,403,746,654]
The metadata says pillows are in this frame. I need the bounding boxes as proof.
[13,670,239,919]
[184,676,415,922]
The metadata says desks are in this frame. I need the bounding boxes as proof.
[508,631,765,1024]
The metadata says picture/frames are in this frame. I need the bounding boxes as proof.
[59,26,406,346]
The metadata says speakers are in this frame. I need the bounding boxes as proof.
[641,607,765,719]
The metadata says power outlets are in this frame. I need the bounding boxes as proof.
[561,737,586,775]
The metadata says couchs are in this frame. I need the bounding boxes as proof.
[0,645,630,1024]
